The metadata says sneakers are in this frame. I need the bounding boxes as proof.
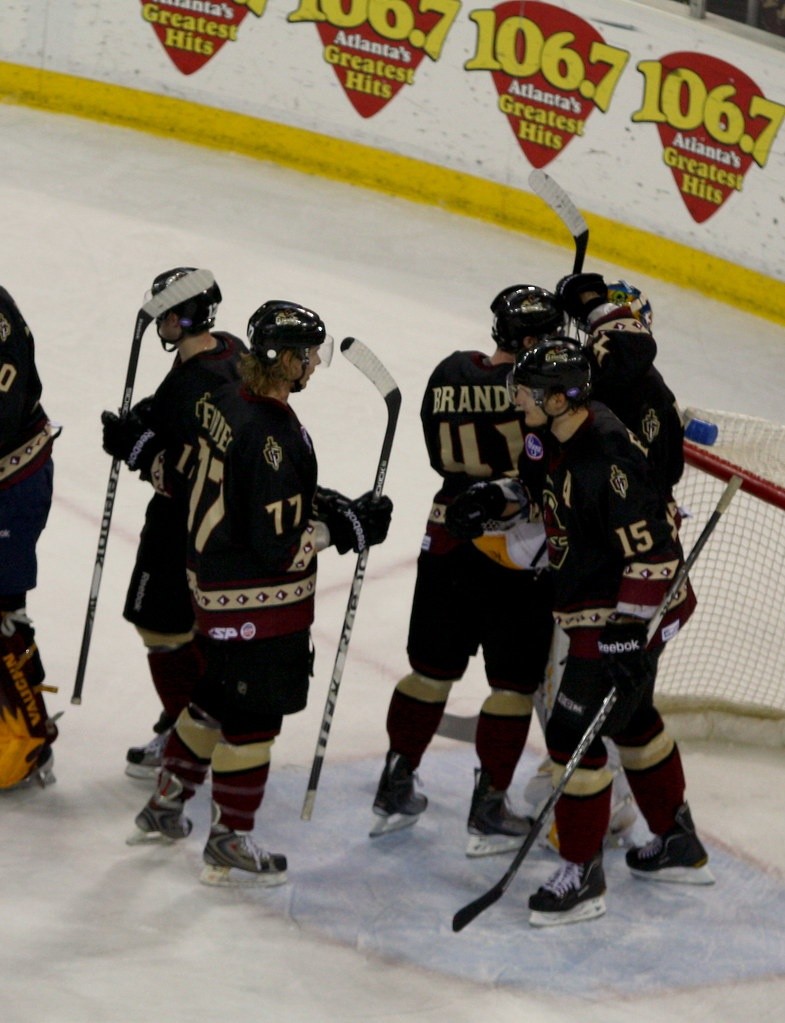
[127,798,193,846]
[465,788,537,857]
[124,711,177,790]
[199,826,287,887]
[528,856,606,926]
[524,757,566,804]
[368,772,427,836]
[626,800,716,883]
[536,789,650,853]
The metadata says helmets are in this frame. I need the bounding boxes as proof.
[608,280,652,325]
[247,300,325,365]
[513,338,592,405]
[152,268,222,344]
[556,273,608,317]
[490,285,565,351]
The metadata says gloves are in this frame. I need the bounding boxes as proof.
[324,491,394,555]
[101,408,164,471]
[598,620,647,684]
[312,488,353,522]
[445,481,507,542]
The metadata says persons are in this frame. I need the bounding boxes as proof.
[523,273,719,853]
[0,285,62,791]
[101,266,249,777]
[126,300,393,888]
[445,336,717,925]
[370,284,571,857]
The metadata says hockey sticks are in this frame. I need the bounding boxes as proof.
[452,475,742,932]
[528,167,589,274]
[70,269,215,705]
[300,337,403,822]
